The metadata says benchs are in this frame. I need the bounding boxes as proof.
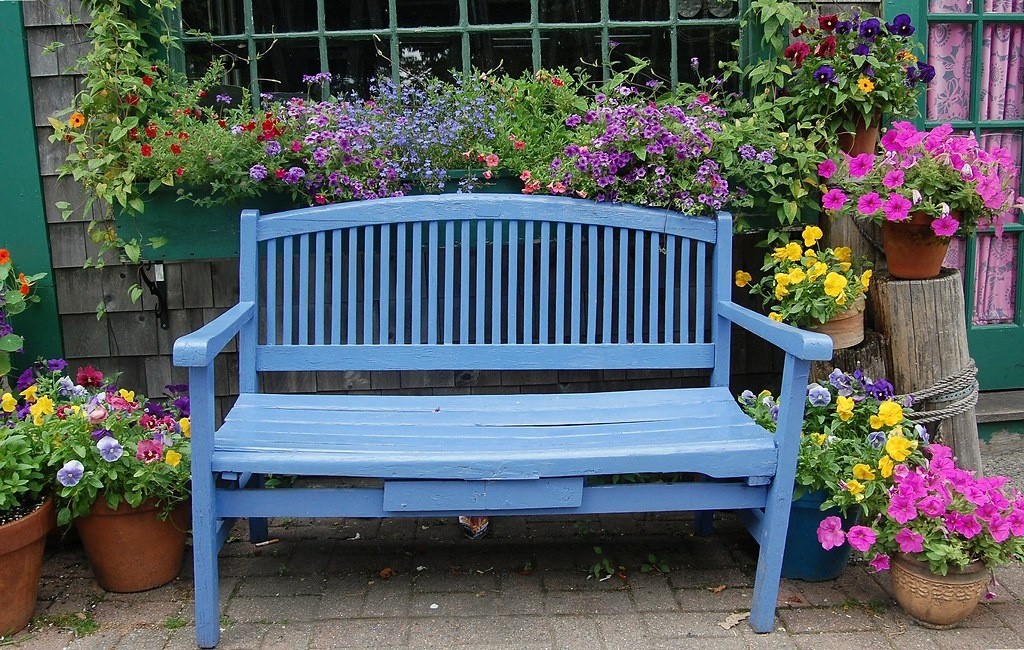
[172,192,835,650]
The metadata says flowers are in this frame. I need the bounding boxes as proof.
[1,357,73,518]
[816,442,1024,600]
[737,366,930,518]
[38,1,844,324]
[784,1,936,131]
[0,248,48,406]
[817,120,1024,242]
[736,222,876,327]
[43,362,191,546]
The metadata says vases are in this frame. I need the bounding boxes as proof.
[819,107,882,158]
[0,489,54,639]
[73,489,190,593]
[780,488,860,582]
[888,548,990,630]
[808,297,865,350]
[881,211,961,279]
